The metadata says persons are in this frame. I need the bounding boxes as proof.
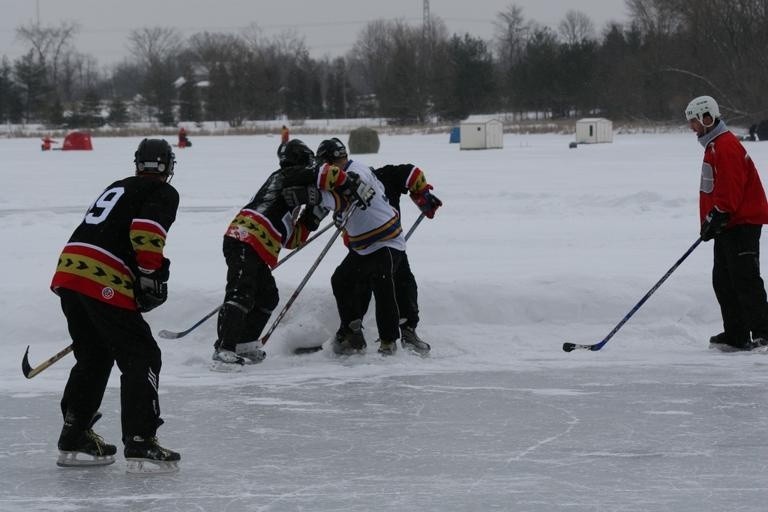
[282,138,405,358]
[282,125,289,143]
[178,127,188,148]
[209,138,360,372]
[684,95,768,351]
[50,138,182,474]
[41,136,52,150]
[748,124,758,141]
[331,125,441,356]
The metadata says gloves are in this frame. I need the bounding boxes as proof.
[129,257,173,315]
[281,180,322,209]
[300,204,330,231]
[699,203,731,243]
[342,170,376,212]
[409,183,443,219]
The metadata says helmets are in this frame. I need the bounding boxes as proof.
[315,136,348,163]
[276,138,315,169]
[683,94,725,130]
[133,138,177,183]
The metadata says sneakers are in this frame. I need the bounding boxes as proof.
[236,347,266,366]
[209,350,245,373]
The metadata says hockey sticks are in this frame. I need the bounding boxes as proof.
[21,343,75,379]
[157,222,337,339]
[293,210,426,355]
[562,235,703,353]
[236,201,360,354]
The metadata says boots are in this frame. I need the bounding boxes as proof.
[743,336,768,354]
[709,331,749,355]
[329,323,371,358]
[56,409,117,466]
[399,328,431,359]
[376,339,398,358]
[122,418,182,474]
